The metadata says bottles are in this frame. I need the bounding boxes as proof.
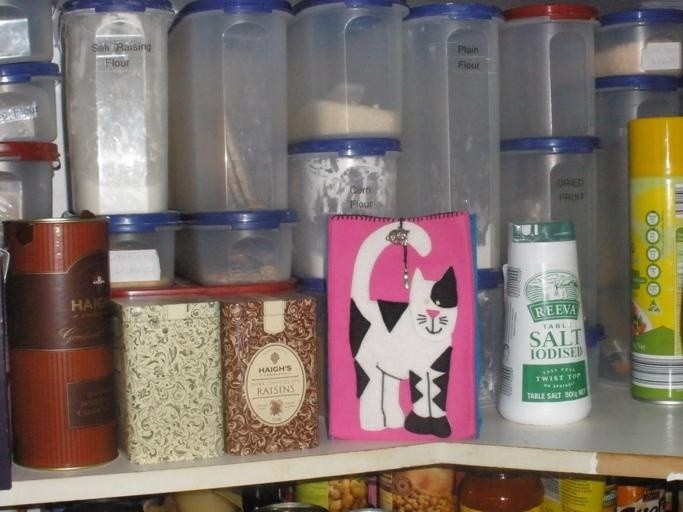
[630,117,683,407]
[497,218,592,427]
